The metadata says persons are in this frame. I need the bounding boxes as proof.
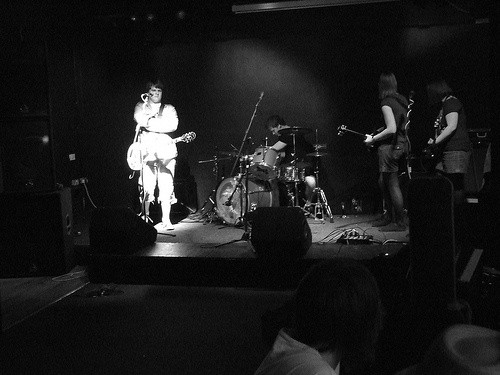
[254,258,380,375]
[363,70,412,232]
[427,72,473,175]
[265,114,319,213]
[134,80,179,230]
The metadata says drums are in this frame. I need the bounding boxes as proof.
[251,146,280,180]
[278,166,306,183]
[215,172,273,226]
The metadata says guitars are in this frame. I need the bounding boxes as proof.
[338,124,386,153]
[126,132,197,170]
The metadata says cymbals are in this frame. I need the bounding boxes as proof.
[278,126,313,137]
[307,152,330,157]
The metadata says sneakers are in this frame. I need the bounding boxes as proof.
[372,217,393,227]
[380,222,406,231]
